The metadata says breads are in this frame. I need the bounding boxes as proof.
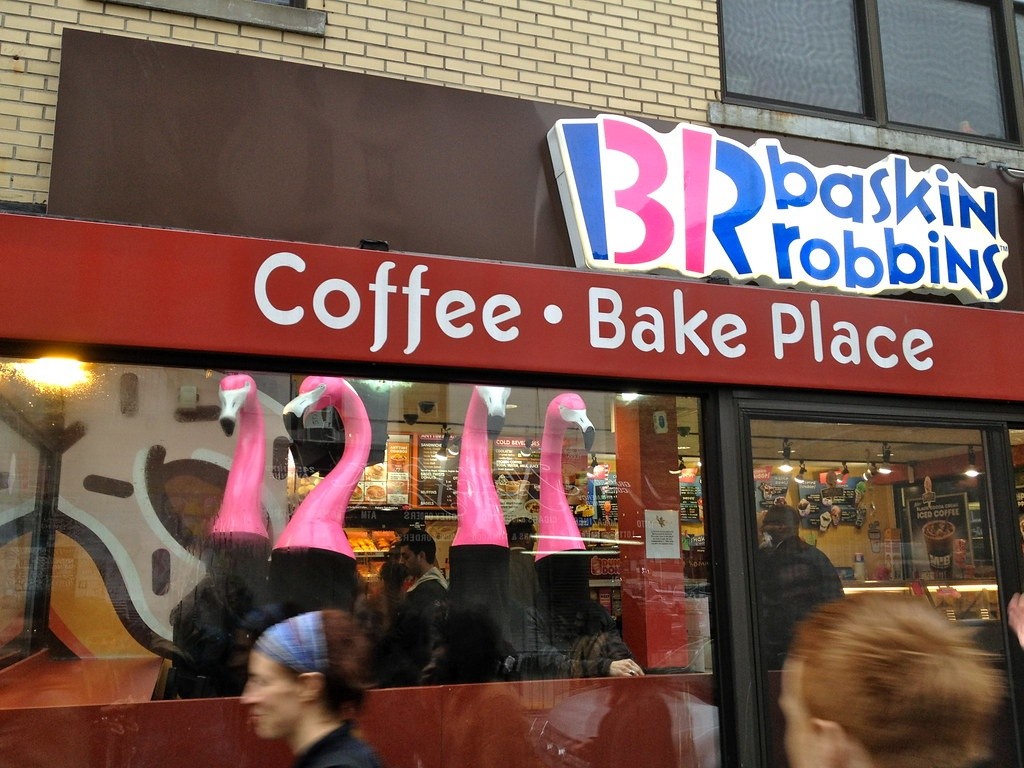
[297,476,324,496]
[352,465,385,499]
[347,530,396,580]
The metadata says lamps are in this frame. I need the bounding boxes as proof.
[436,432,450,461]
[878,449,893,473]
[863,469,878,481]
[837,467,849,484]
[679,462,686,478]
[778,446,793,472]
[965,450,977,476]
[795,465,807,483]
[449,438,461,456]
[521,440,532,457]
[591,457,598,468]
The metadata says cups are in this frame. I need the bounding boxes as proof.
[922,520,955,580]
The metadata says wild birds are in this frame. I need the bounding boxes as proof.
[211,372,270,551]
[450,385,514,552]
[278,375,373,560]
[531,391,595,563]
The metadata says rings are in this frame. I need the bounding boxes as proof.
[630,670,635,675]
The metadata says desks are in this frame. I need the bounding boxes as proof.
[0,649,165,710]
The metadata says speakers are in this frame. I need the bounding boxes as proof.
[285,378,393,475]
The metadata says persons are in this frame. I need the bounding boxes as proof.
[778,590,1007,768]
[754,503,845,670]
[241,609,385,768]
[166,517,645,699]
